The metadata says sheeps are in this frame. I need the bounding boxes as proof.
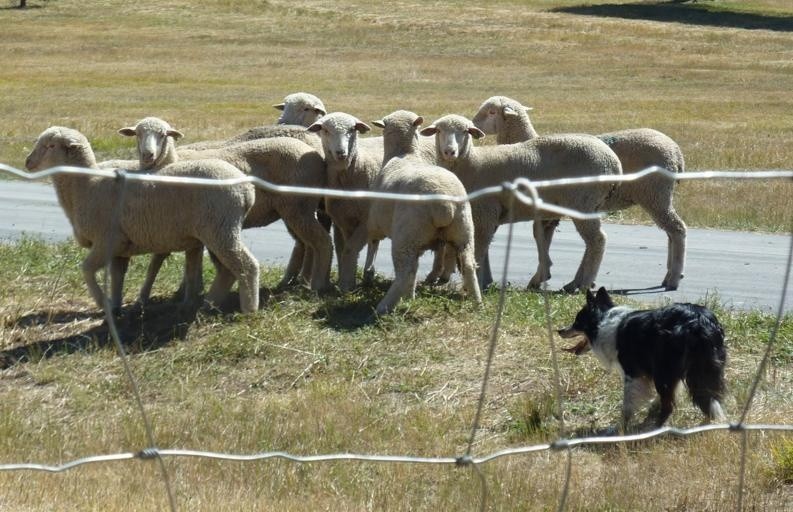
[23,89,688,321]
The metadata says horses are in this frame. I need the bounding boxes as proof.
[556,283,729,426]
